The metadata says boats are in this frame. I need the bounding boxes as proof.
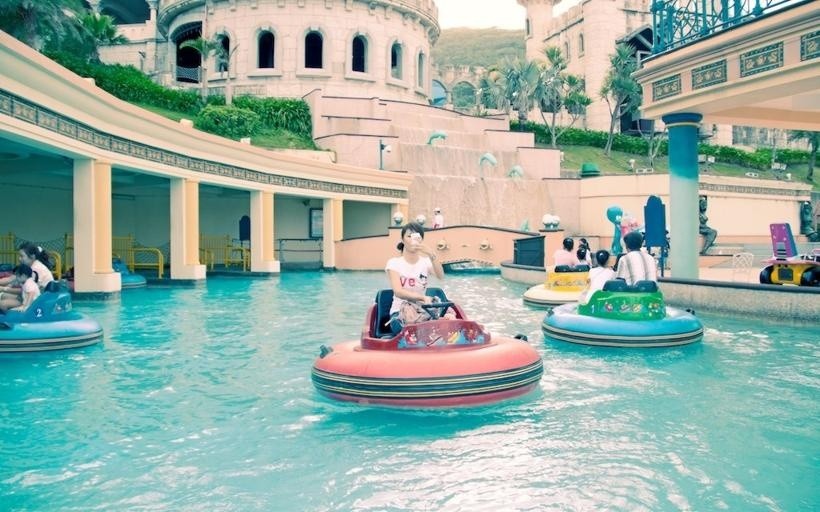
[0,278,104,351]
[112,253,148,287]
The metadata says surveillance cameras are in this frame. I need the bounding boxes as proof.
[384,145,393,153]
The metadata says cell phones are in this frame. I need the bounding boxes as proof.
[411,232,420,244]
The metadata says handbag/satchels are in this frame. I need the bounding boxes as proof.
[398,295,440,327]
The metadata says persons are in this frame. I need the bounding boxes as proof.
[10,264,41,312]
[699,199,716,256]
[801,202,817,242]
[0,242,54,311]
[385,222,457,337]
[432,207,443,228]
[553,232,657,304]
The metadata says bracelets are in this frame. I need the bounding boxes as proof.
[430,255,437,260]
[4,286,9,292]
